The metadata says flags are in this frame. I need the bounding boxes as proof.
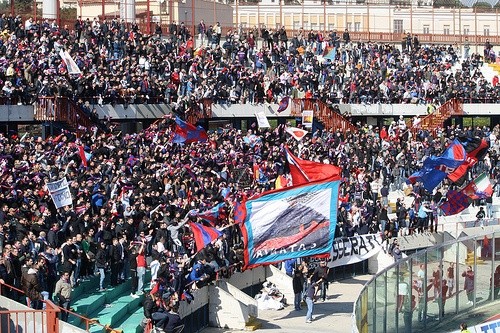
[243,179,340,270]
[406,139,466,195]
[437,189,472,216]
[442,137,490,183]
[461,171,492,199]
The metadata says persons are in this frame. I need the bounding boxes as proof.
[0,0,500,333]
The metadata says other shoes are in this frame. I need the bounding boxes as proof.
[435,318,439,320]
[433,299,436,301]
[309,318,314,320]
[467,301,471,304]
[322,300,324,302]
[83,276,90,279]
[130,293,140,298]
[471,301,473,305]
[306,321,311,323]
[90,275,94,278]
